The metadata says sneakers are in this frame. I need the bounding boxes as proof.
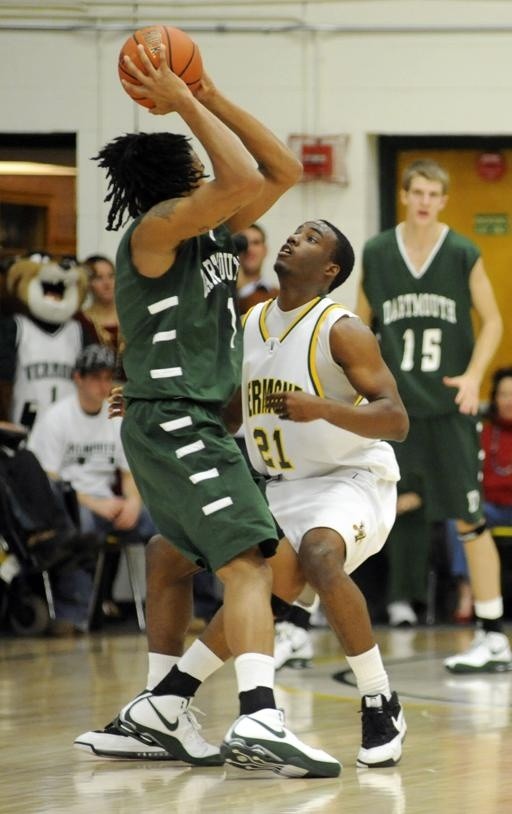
[386,600,418,627]
[274,620,314,670]
[443,628,512,673]
[356,692,408,768]
[74,690,342,779]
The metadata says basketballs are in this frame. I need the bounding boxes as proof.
[117,23,202,107]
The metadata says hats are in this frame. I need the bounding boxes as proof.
[75,343,116,374]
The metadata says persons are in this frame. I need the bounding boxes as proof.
[91,38,340,778]
[273,156,512,672]
[0,215,512,636]
[75,215,410,770]
